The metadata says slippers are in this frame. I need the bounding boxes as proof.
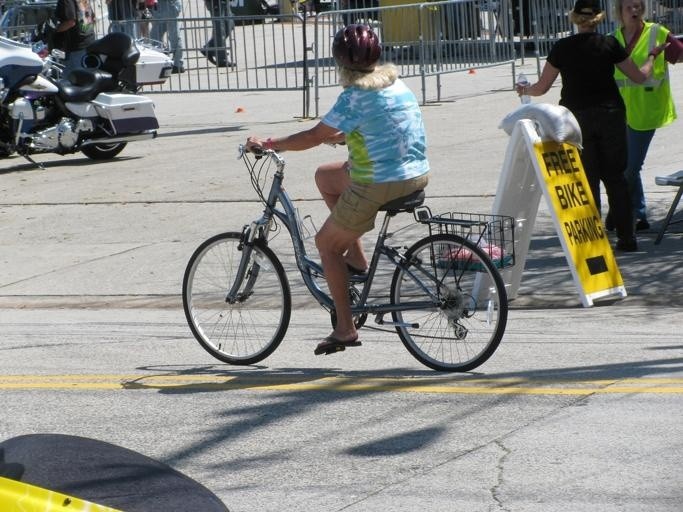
[314,337,362,355]
[347,263,369,273]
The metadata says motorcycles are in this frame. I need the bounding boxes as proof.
[0,18,176,170]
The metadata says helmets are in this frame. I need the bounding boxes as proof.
[333,24,382,68]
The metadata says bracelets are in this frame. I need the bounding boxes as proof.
[267,137,274,149]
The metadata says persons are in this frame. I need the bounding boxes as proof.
[246,25,430,355]
[105,0,185,74]
[48,0,95,60]
[200,0,236,67]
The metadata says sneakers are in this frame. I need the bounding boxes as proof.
[606,216,650,251]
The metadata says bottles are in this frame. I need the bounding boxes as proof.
[516,71,530,105]
[32,39,48,54]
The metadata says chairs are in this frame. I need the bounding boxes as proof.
[58,68,113,103]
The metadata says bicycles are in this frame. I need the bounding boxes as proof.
[180,134,518,374]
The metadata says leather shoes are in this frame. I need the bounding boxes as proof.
[202,48,236,67]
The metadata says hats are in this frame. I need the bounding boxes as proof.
[574,0,601,15]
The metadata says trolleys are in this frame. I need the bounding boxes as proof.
[377,1,448,60]
